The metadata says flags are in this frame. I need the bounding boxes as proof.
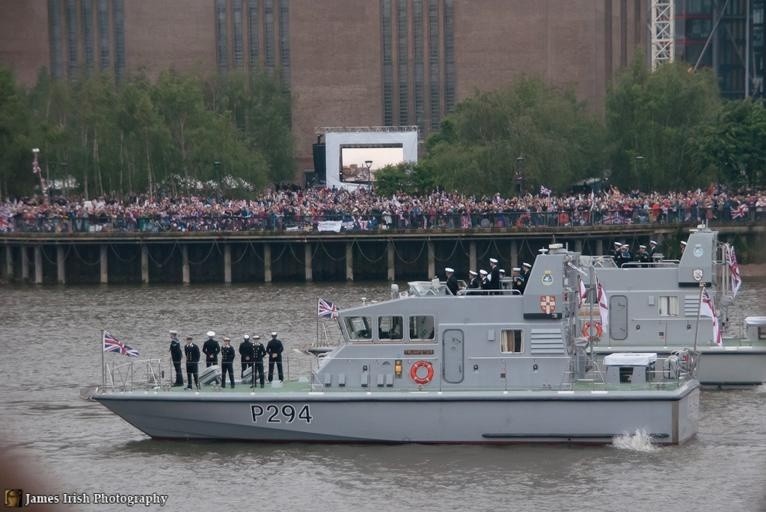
[700,289,723,345]
[103,330,139,358]
[726,245,742,297]
[595,276,609,332]
[318,298,339,320]
[578,277,587,306]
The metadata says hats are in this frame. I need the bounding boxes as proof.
[444,257,532,276]
[613,240,688,250]
[168,329,278,342]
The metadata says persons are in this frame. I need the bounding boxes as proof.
[613,240,658,268]
[239,334,253,378]
[169,330,183,386]
[184,336,200,390]
[466,256,532,295]
[266,331,283,384]
[248,335,266,388]
[220,337,235,389]
[680,240,686,256]
[445,267,459,295]
[203,330,220,384]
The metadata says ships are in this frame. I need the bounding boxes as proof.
[76,243,700,446]
[301,222,764,392]
